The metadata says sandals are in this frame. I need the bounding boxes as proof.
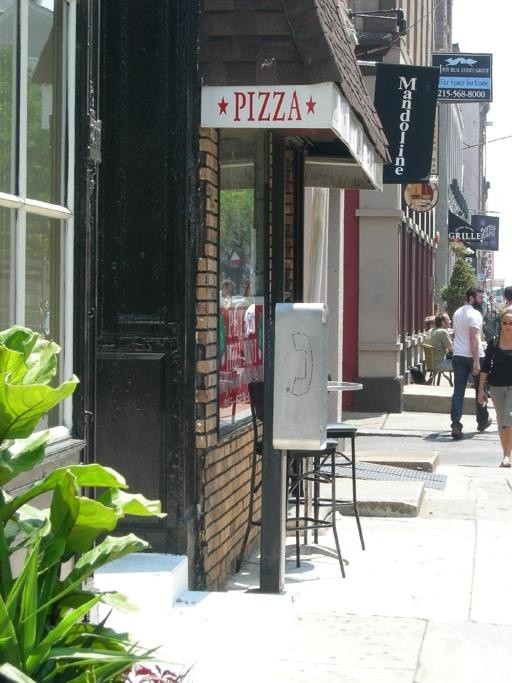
[501,456,512,467]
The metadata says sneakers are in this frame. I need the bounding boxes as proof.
[452,422,464,439]
[478,418,493,431]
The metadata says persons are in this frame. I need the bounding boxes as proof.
[425,286,512,468]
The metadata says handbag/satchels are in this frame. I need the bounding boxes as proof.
[489,336,497,379]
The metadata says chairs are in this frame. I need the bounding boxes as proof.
[422,342,455,386]
[235,380,347,578]
[301,426,365,549]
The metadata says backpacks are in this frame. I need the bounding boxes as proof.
[411,368,425,384]
